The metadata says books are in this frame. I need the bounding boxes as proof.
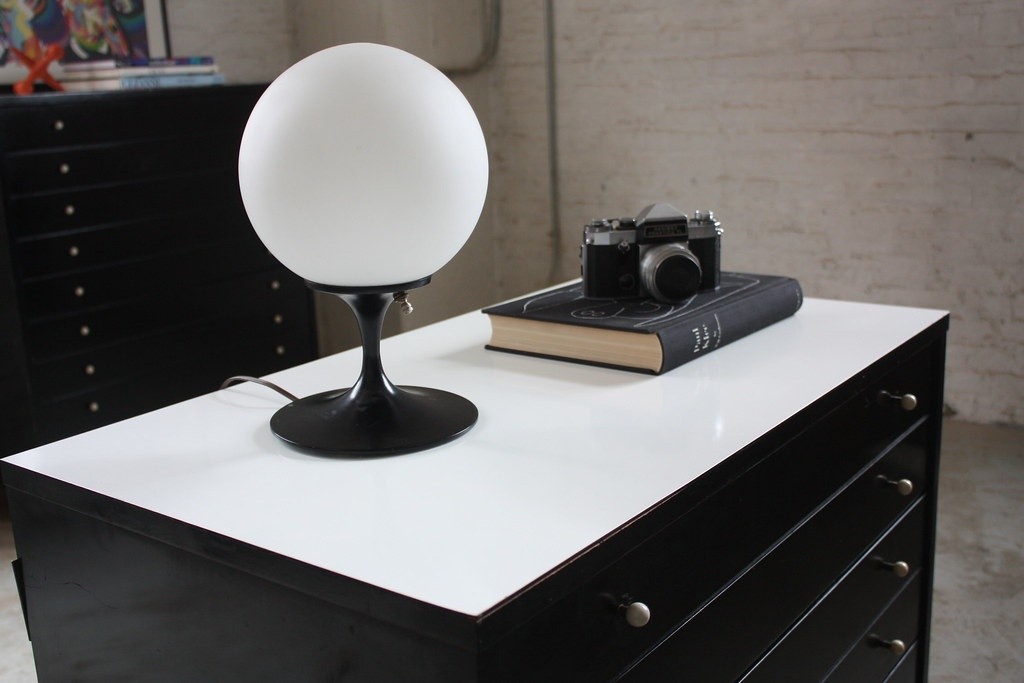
[63,52,226,93]
[479,264,804,376]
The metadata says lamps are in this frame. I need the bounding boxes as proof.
[236,40,489,461]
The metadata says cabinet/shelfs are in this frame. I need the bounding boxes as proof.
[0,80,318,521]
[0,273,951,683]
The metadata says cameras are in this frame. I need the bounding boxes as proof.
[579,202,724,304]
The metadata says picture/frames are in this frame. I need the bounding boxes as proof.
[0,0,172,72]
